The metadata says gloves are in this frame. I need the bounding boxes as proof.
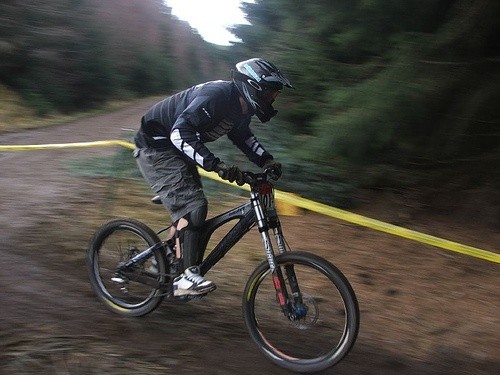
[263,159,282,181]
[213,158,245,186]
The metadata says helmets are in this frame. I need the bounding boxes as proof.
[232,57,295,123]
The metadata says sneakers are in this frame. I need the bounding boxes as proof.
[172,265,215,296]
[151,256,179,273]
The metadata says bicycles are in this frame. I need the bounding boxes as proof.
[84,164,360,373]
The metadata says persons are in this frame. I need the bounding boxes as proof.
[132,57,297,298]
